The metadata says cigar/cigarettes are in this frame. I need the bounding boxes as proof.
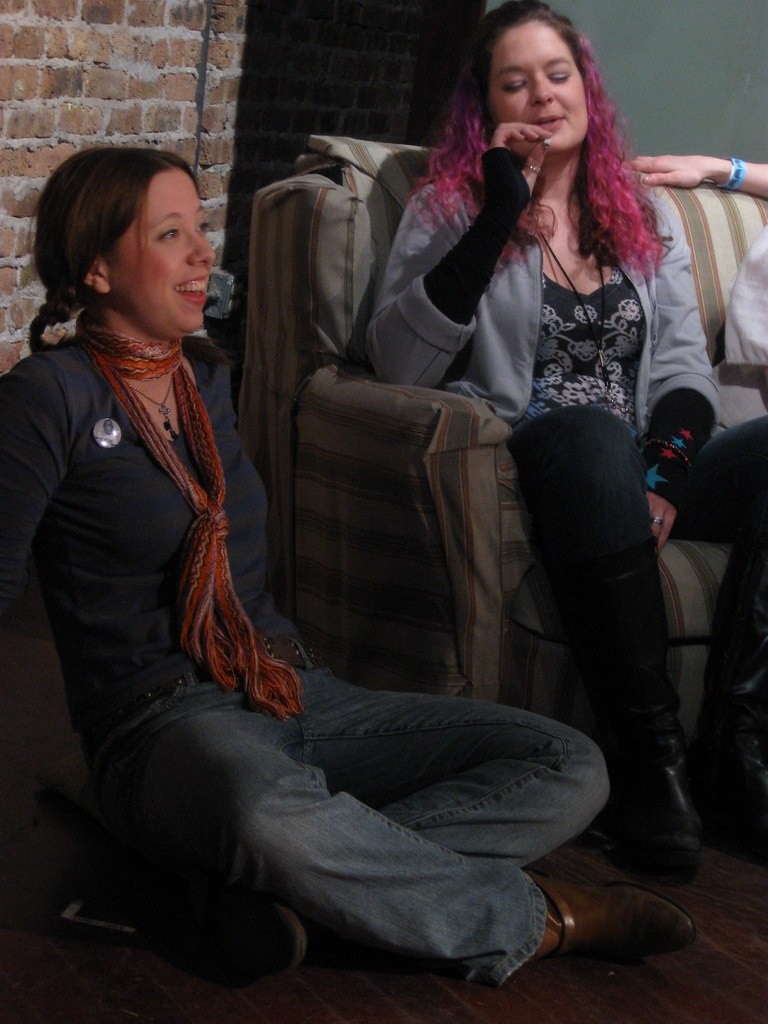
[543,139,549,146]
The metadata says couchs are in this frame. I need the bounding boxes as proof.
[238,132,767,749]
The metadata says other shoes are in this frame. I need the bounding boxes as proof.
[175,878,308,979]
[525,869,698,960]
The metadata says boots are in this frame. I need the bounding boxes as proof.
[544,533,704,869]
[701,485,768,859]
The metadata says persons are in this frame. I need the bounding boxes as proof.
[367,0,768,878]
[0,148,699,978]
[94,420,117,447]
[619,155,768,382]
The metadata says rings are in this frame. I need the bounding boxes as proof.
[653,515,663,524]
[528,164,541,172]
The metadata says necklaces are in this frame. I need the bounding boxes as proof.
[129,374,178,439]
[540,230,629,414]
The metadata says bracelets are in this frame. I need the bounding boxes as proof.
[715,157,746,190]
[644,439,692,469]
